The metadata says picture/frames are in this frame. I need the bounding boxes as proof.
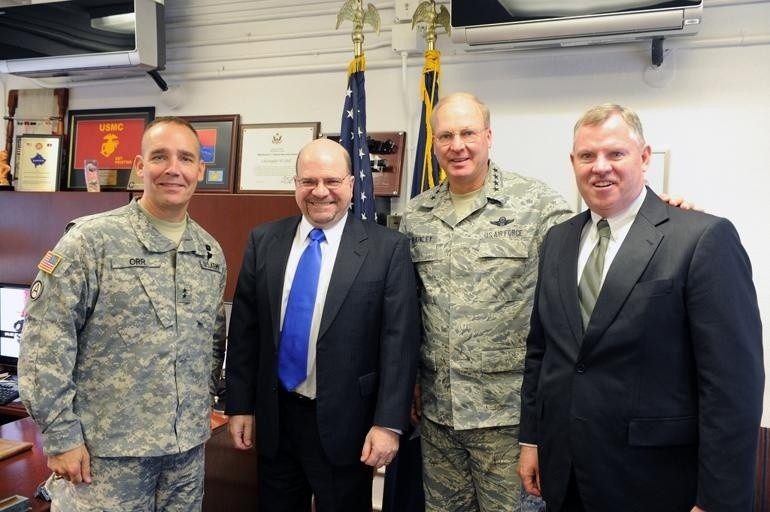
[175,113,241,195]
[235,122,321,195]
[60,106,155,192]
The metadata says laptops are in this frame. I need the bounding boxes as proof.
[0,282,31,408]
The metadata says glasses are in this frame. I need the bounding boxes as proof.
[433,126,486,147]
[296,171,351,190]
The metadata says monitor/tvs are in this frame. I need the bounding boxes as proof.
[1,0,165,81]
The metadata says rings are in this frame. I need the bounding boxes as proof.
[54,473,63,480]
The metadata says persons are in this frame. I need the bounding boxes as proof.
[517,100,766,512]
[224,138,422,512]
[18,116,229,512]
[397,91,705,512]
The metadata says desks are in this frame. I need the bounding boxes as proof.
[0,416,50,512]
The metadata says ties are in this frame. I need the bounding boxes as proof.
[578,220,611,337]
[277,228,326,392]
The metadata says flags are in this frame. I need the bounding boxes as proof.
[338,51,378,224]
[37,250,62,274]
[409,48,447,201]
